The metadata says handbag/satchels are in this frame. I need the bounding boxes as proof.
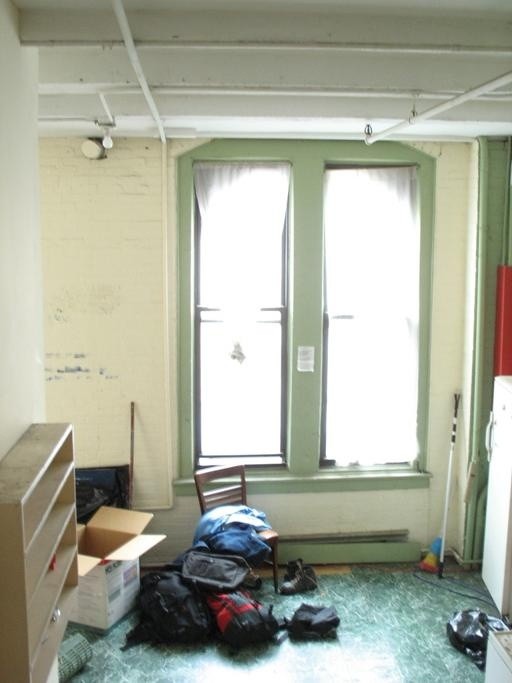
[182,551,262,593]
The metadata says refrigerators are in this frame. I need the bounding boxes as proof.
[478,374,512,619]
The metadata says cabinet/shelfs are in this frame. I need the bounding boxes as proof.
[0,421,80,682]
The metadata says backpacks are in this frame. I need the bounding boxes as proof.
[206,588,280,648]
[138,567,213,645]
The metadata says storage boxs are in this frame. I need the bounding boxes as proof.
[68,505,169,631]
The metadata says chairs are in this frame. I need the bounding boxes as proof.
[191,461,282,595]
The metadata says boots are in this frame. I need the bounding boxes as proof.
[280,566,318,595]
[284,559,303,578]
